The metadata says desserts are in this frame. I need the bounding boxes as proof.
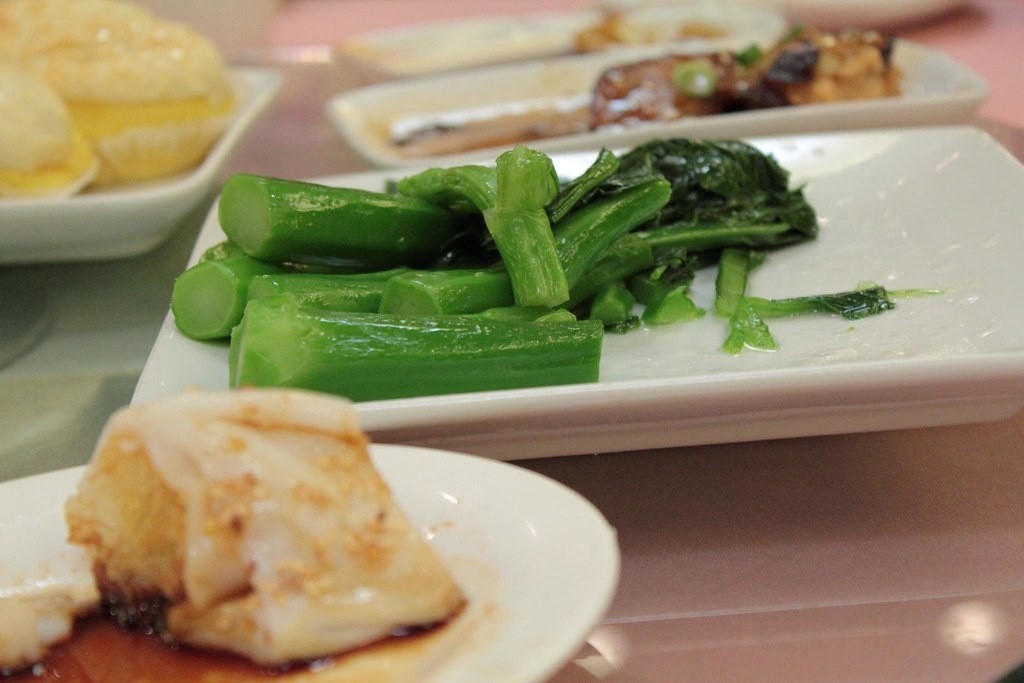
[59,385,466,668]
[0,1,234,199]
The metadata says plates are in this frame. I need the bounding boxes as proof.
[324,0,989,166]
[1,68,284,261]
[129,125,1023,459]
[1,445,621,682]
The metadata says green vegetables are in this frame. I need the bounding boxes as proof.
[169,135,895,402]
